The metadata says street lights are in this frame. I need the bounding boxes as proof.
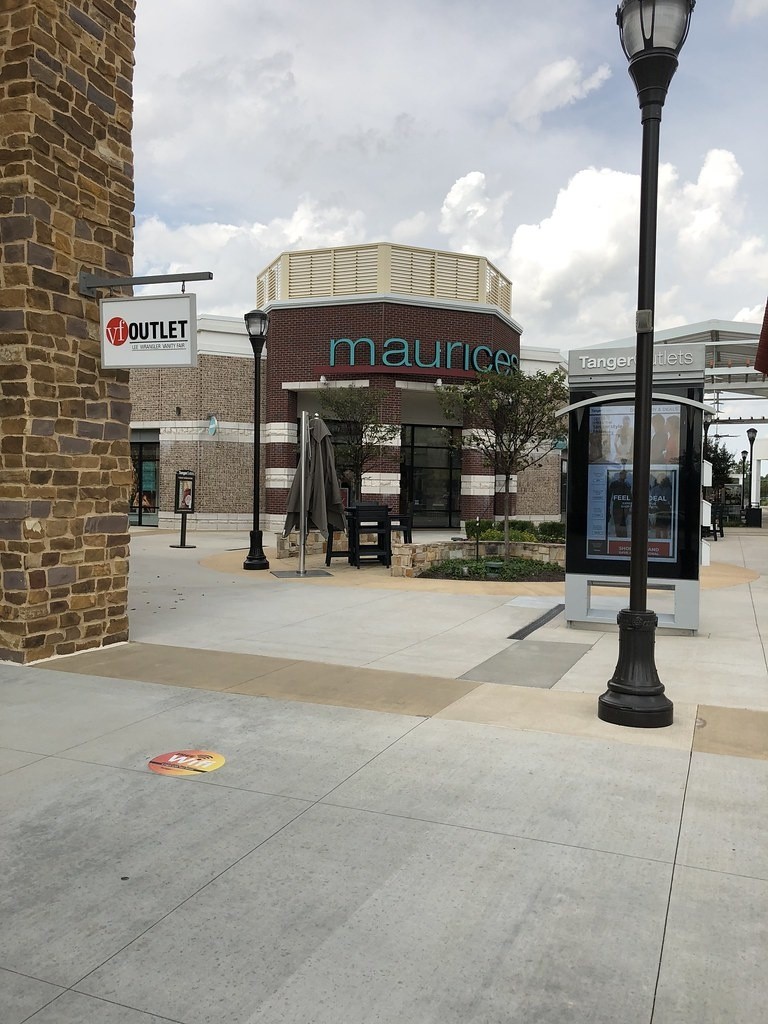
[747,427,757,508]
[621,459,626,471]
[593,0,693,730]
[740,451,748,516]
[241,309,270,569]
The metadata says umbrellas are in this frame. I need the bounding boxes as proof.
[282,412,346,574]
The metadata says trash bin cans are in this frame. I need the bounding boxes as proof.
[745,508,762,528]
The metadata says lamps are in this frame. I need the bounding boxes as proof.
[320,376,328,385]
[434,379,442,387]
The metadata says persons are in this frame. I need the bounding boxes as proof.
[653,478,673,539]
[607,470,632,538]
